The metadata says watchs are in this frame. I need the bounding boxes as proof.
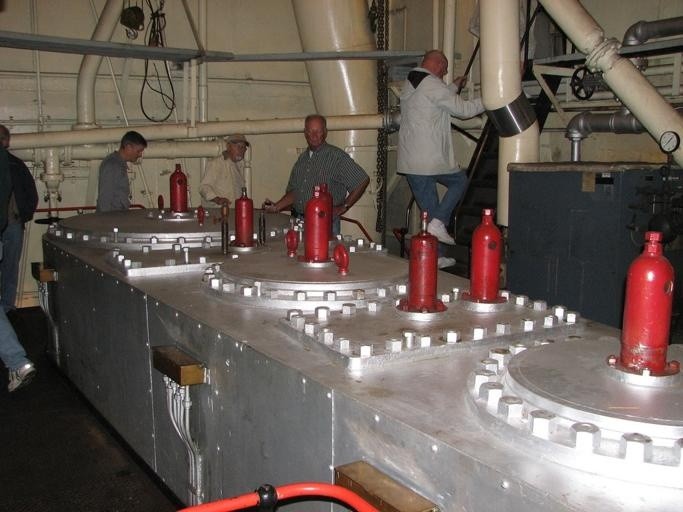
[342,201,348,210]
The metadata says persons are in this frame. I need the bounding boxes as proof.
[0,146,37,396]
[0,125,38,316]
[260,113,370,236]
[196,131,250,208]
[394,48,486,272]
[93,130,147,213]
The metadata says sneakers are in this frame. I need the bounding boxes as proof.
[8,363,35,393]
[438,256,455,270]
[427,223,455,245]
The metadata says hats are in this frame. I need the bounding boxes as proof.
[224,133,250,148]
